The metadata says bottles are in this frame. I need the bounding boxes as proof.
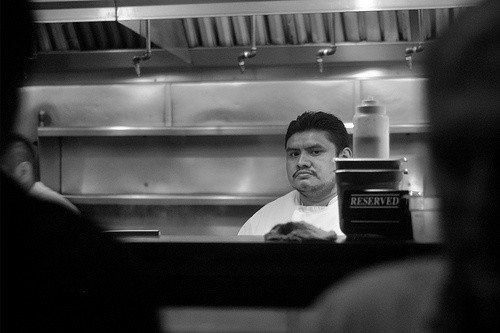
[353,97,390,160]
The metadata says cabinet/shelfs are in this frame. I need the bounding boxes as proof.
[35,110,444,233]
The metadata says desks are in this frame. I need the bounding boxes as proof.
[111,229,445,245]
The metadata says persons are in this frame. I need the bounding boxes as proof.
[238,111,353,242]
[296,1,500,333]
[0,0,164,333]
[2,133,81,216]
